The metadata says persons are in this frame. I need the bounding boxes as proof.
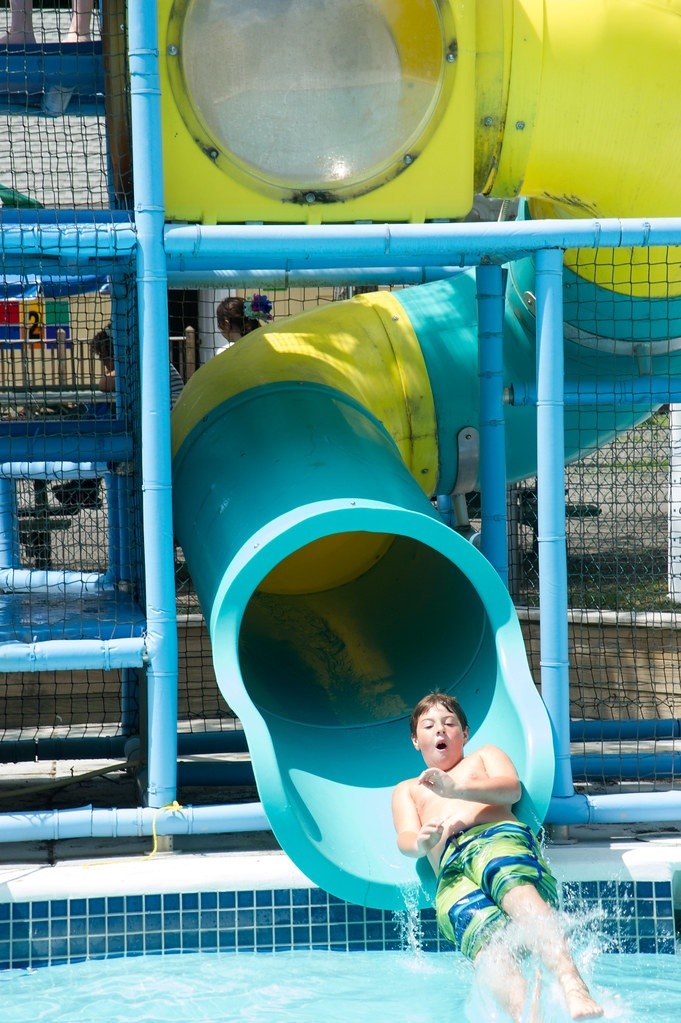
[216,293,273,343]
[88,323,184,411]
[391,694,603,1023]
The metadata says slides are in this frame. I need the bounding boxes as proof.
[152,4,679,918]
